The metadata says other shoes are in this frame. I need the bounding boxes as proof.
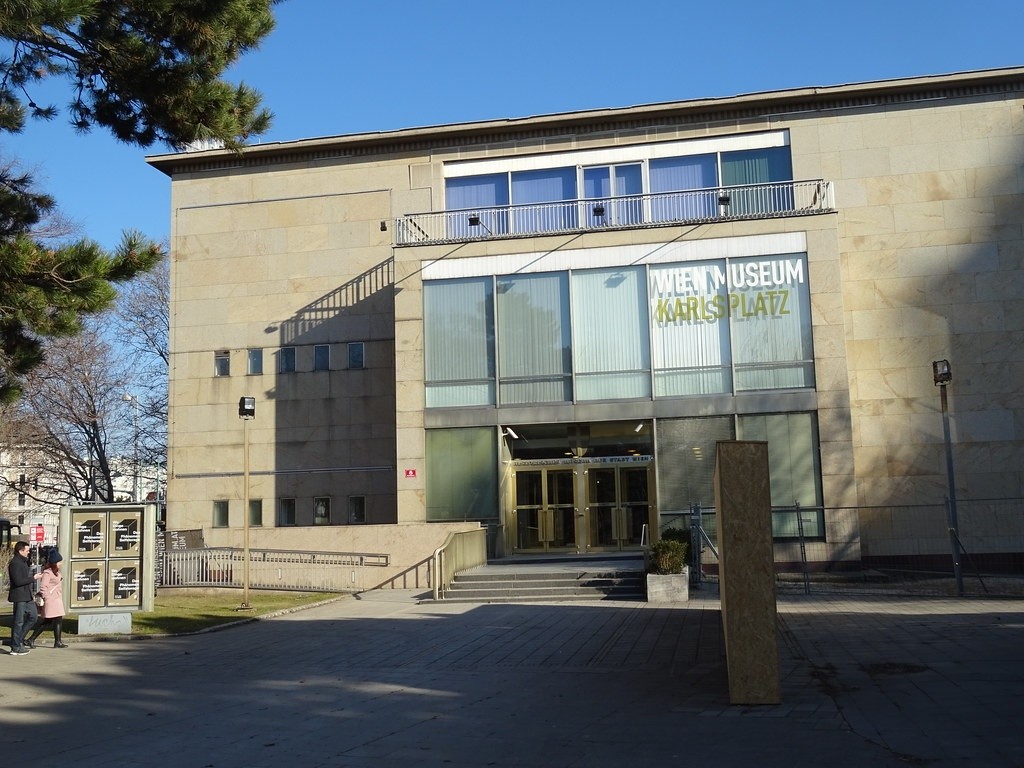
[54,641,68,648]
[10,647,30,656]
[24,638,36,649]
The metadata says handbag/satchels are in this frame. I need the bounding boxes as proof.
[35,593,44,607]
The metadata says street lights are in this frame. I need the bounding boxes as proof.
[121,392,140,505]
[932,360,967,596]
[238,397,255,613]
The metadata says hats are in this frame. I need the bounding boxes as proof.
[49,549,62,563]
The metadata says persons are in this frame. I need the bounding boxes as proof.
[24,550,68,649]
[8,540,42,655]
